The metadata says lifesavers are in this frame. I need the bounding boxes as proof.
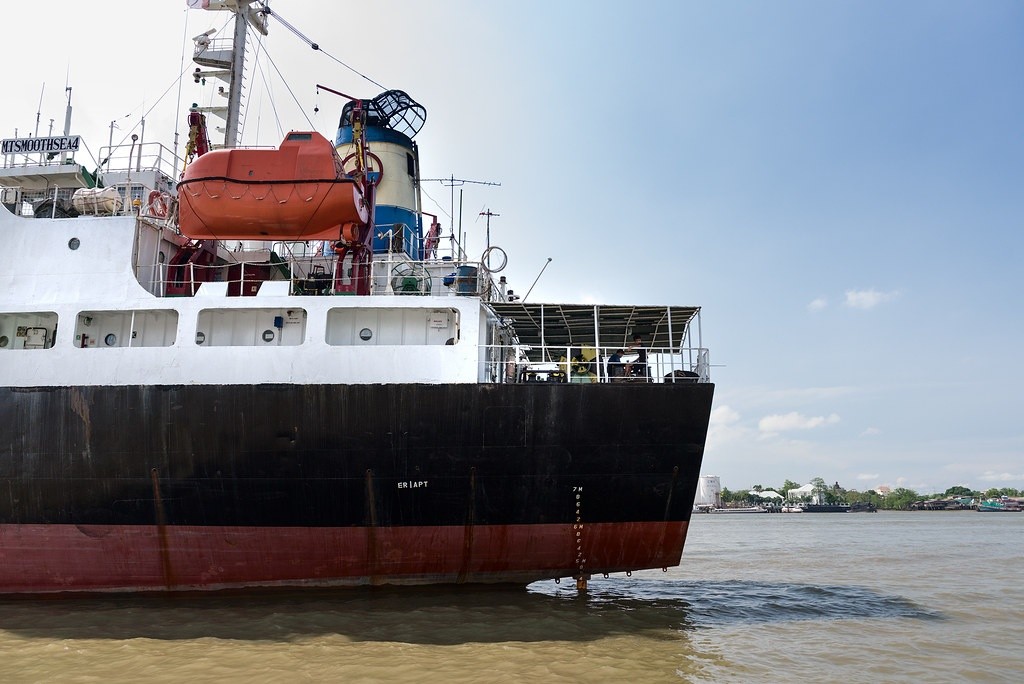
[147,190,167,217]
[329,235,348,253]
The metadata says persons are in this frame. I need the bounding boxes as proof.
[607,336,648,383]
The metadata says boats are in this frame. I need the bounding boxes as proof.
[781,503,852,513]
[910,495,1024,512]
[1,0,716,603]
[697,505,769,513]
